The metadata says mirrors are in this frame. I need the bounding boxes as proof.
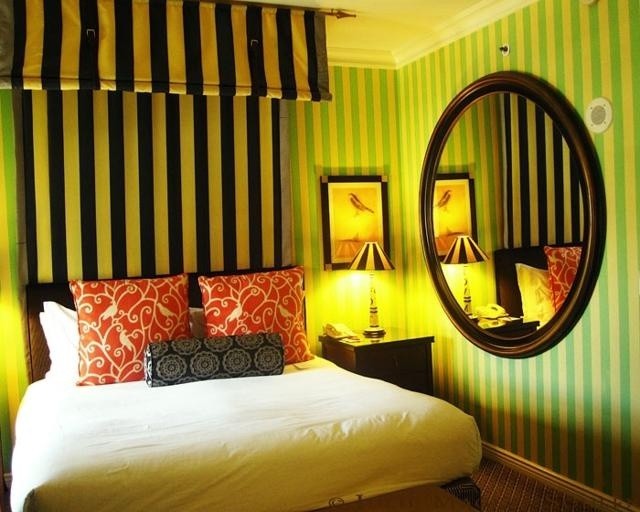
[417,70,606,360]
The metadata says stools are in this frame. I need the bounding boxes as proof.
[311,485,481,512]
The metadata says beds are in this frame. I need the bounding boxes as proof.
[10,265,482,511]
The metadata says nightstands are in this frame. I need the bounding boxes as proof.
[478,315,540,337]
[318,332,437,396]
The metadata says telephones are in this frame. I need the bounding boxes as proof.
[322,322,354,339]
[475,303,509,318]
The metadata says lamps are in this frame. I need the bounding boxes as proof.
[442,235,489,321]
[348,241,394,337]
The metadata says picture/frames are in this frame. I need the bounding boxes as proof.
[432,172,481,261]
[320,173,391,276]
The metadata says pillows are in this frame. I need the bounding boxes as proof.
[514,263,556,325]
[499,267,523,317]
[543,244,582,312]
[141,331,286,387]
[69,274,194,386]
[197,265,315,363]
[43,300,204,382]
